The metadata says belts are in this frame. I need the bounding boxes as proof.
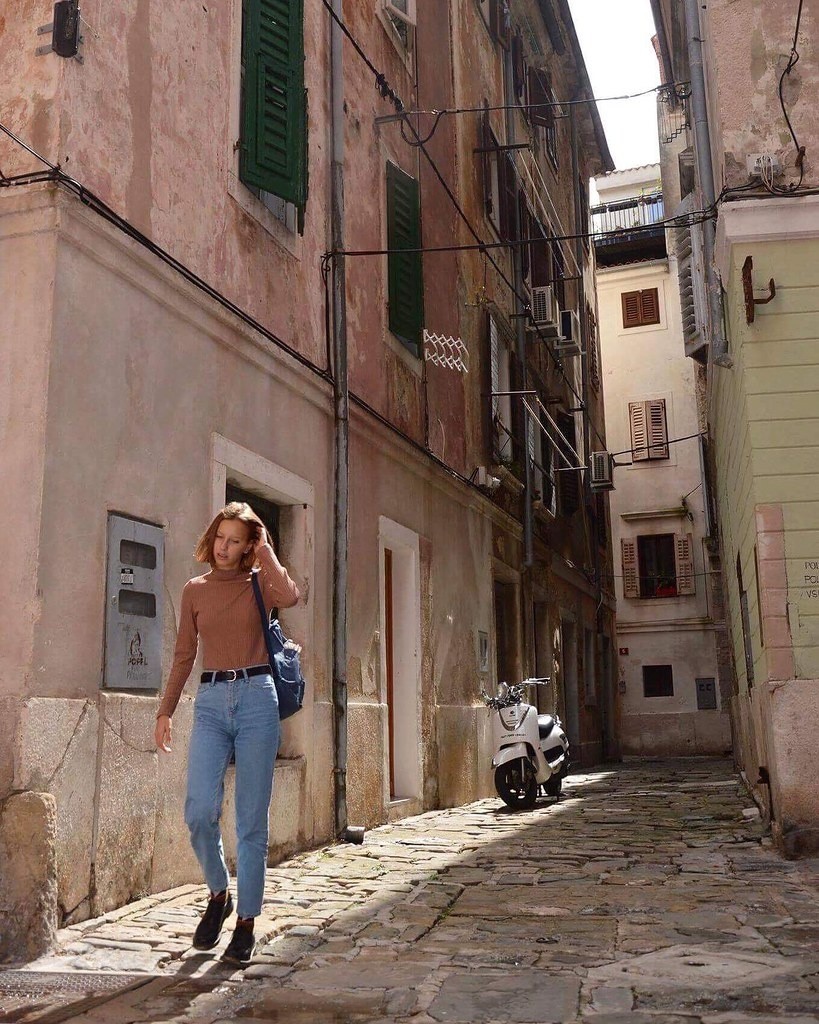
[199,663,272,684]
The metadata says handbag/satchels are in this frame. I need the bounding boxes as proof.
[251,567,305,721]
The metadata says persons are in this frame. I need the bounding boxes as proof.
[153,501,301,967]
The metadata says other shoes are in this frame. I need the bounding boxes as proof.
[194,888,234,951]
[219,930,257,968]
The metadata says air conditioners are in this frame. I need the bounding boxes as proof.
[529,286,559,337]
[559,309,582,353]
[589,451,614,487]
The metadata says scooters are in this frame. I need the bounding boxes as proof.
[481,675,569,809]
